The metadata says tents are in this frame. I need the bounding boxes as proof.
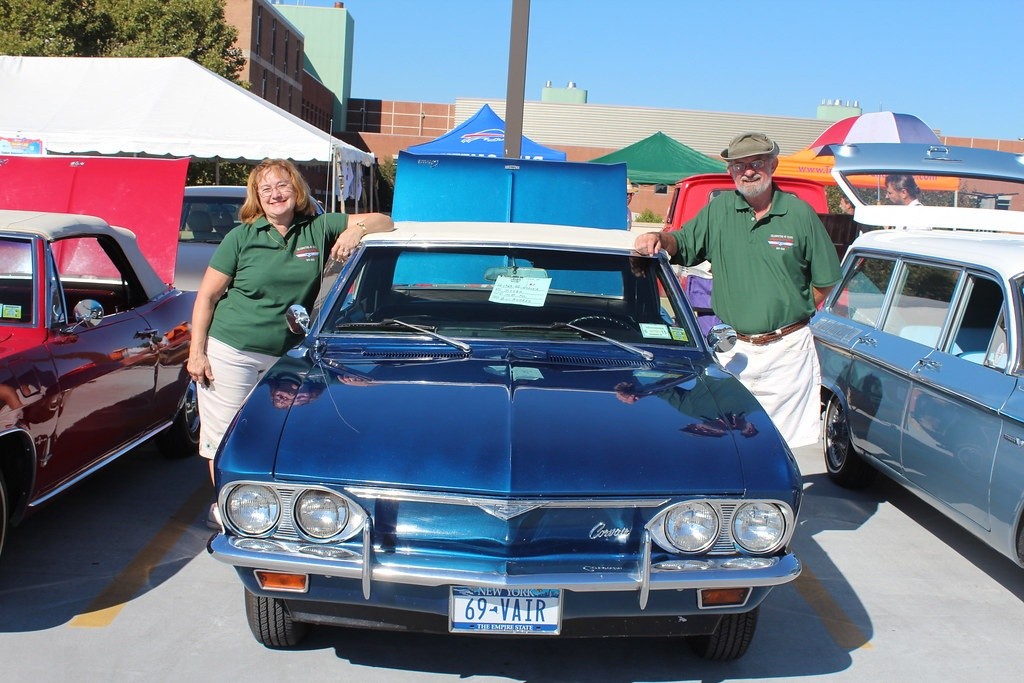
[770,146,961,208]
[585,129,728,185]
[406,104,567,162]
[0,56,376,215]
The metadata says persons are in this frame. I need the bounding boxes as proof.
[613,373,760,439]
[839,193,869,236]
[635,131,844,448]
[877,172,932,230]
[184,158,395,529]
[162,332,169,347]
[258,354,369,409]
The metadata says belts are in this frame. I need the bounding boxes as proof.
[736,316,810,344]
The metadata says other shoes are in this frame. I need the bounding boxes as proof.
[206,500,222,530]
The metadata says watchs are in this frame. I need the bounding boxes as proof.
[356,222,368,234]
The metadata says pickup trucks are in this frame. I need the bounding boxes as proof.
[654,173,850,318]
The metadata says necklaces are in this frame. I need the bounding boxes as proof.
[267,229,290,249]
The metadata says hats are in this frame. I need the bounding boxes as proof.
[719,132,780,162]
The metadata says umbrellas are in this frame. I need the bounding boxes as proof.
[808,111,942,202]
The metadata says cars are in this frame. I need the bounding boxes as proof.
[0,152,200,557]
[175,185,325,291]
[207,152,803,663]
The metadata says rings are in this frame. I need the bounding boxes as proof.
[351,380,354,381]
[344,250,349,253]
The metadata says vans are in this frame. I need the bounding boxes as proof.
[804,142,1023,574]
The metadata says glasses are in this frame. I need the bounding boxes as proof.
[256,181,296,196]
[728,158,772,173]
